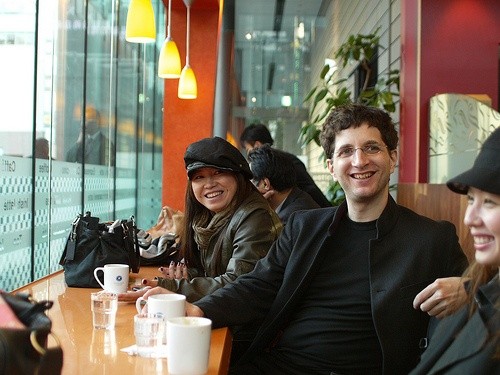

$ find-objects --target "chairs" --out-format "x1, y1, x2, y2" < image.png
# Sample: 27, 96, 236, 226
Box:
396, 181, 482, 266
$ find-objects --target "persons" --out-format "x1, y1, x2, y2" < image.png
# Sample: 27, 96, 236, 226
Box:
143, 105, 476, 375
238, 122, 334, 209
65, 108, 113, 165
246, 143, 322, 225
408, 126, 500, 375
26, 138, 56, 160
95, 136, 285, 303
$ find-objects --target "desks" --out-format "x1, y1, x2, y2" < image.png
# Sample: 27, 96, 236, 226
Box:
0, 224, 234, 375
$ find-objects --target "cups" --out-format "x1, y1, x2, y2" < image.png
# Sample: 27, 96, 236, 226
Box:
134, 314, 163, 355
166, 317, 212, 375
90, 293, 118, 329
94, 264, 129, 294
136, 294, 186, 344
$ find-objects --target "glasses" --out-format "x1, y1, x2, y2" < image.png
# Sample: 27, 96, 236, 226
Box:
333, 143, 392, 156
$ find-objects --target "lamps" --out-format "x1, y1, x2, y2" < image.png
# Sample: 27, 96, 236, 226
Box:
159, 0, 182, 80
126, 0, 156, 44
178, 0, 198, 99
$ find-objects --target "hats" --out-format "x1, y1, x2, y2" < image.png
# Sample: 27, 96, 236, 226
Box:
446, 125, 500, 196
183, 137, 254, 182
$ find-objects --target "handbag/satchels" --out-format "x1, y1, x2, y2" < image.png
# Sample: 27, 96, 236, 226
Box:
145, 205, 184, 238
136, 227, 182, 267
0, 289, 65, 375
57, 211, 142, 288
